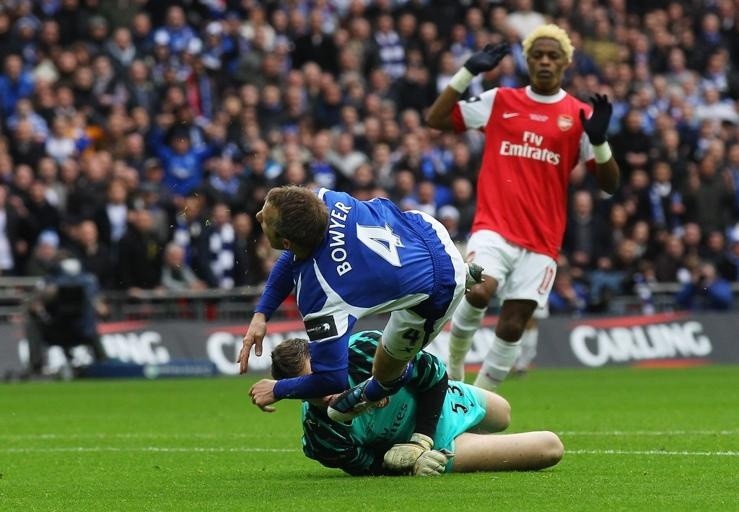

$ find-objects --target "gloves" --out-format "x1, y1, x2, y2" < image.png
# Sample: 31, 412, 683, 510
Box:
578, 92, 614, 146
463, 41, 511, 76
383, 432, 448, 477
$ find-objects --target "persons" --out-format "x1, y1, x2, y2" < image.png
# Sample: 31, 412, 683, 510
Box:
1, 2, 737, 379
231, 22, 622, 475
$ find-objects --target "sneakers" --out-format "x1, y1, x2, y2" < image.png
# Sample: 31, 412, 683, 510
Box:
466, 262, 486, 286
326, 376, 388, 422
